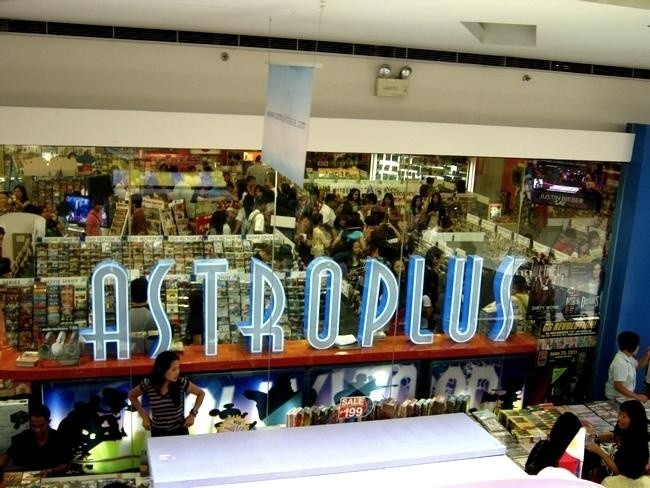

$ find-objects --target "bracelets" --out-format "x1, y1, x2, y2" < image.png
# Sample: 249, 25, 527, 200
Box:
190, 408, 198, 416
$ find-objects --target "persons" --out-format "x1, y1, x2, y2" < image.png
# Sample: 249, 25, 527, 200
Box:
484, 269, 529, 328
0, 185, 72, 237
524, 400, 650, 488
185, 289, 205, 345
131, 194, 148, 235
604, 330, 650, 402
86, 200, 104, 236
553, 227, 603, 262
157, 155, 267, 202
579, 259, 603, 295
129, 277, 157, 353
209, 173, 447, 333
0, 227, 12, 278
128, 351, 205, 436
0, 405, 74, 478
526, 270, 555, 327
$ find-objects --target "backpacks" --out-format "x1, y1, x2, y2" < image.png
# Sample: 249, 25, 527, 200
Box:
241, 212, 262, 234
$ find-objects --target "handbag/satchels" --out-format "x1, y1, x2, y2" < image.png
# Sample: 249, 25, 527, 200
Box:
437, 215, 453, 228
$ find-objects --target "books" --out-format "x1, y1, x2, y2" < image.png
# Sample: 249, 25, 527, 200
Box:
2, 470, 153, 488
472, 395, 650, 470
16, 351, 41, 367
285, 394, 471, 428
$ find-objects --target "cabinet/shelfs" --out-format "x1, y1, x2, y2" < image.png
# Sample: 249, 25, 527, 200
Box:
0, 152, 620, 351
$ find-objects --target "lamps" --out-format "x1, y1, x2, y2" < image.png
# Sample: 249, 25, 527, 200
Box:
373, 62, 411, 99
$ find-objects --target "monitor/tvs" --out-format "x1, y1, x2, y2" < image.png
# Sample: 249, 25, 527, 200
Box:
64, 193, 92, 224
